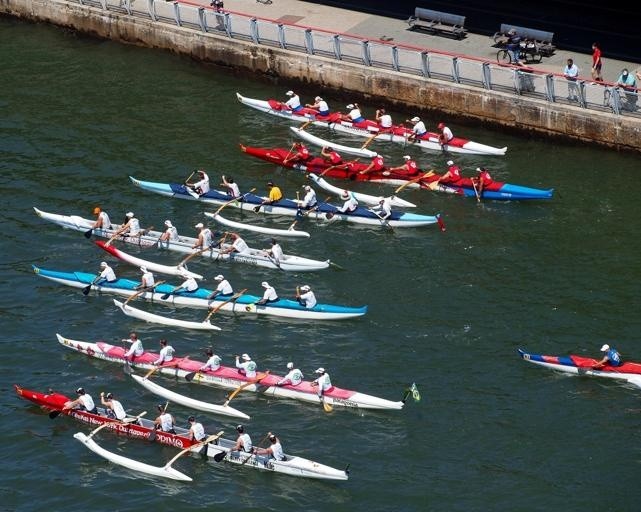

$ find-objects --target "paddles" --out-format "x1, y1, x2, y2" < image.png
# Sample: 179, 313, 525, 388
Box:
170, 164, 404, 240
255, 382, 292, 393
577, 366, 593, 374
123, 341, 133, 376
185, 368, 202, 380
312, 384, 332, 413
79, 270, 301, 326
429, 137, 447, 190
85, 215, 286, 274
49, 399, 269, 473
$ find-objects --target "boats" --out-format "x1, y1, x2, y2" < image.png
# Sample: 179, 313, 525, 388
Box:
237, 93, 508, 159
240, 142, 555, 208
31, 263, 369, 332
34, 207, 330, 280
15, 385, 349, 481
517, 348, 641, 379
129, 175, 441, 239
55, 332, 416, 422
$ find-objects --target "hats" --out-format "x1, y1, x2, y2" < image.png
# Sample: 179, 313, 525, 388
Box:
93, 208, 278, 245
130, 333, 325, 374
265, 180, 385, 202
286, 91, 444, 128
368, 152, 485, 173
508, 29, 516, 35
100, 262, 310, 292
600, 344, 609, 351
76, 388, 276, 441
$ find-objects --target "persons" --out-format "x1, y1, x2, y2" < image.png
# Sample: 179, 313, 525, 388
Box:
591, 343, 623, 370
613, 68, 639, 113
278, 363, 332, 398
200, 349, 256, 377
175, 272, 233, 298
280, 90, 329, 116
93, 261, 154, 290
122, 333, 175, 365
502, 29, 526, 67
263, 181, 358, 213
283, 142, 342, 165
406, 117, 453, 144
62, 387, 126, 420
564, 58, 581, 102
155, 404, 205, 442
185, 170, 242, 202
369, 196, 391, 220
209, 1, 225, 32
230, 425, 284, 462
161, 220, 248, 253
263, 237, 285, 260
439, 161, 493, 194
565, 380, 611, 400
591, 42, 604, 87
341, 103, 393, 128
91, 207, 141, 236
258, 282, 317, 309
360, 152, 417, 175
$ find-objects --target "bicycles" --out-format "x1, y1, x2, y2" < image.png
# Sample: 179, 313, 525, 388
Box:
497, 41, 543, 64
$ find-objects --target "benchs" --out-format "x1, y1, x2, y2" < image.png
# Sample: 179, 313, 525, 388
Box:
492, 22, 555, 57
403, 6, 468, 39
